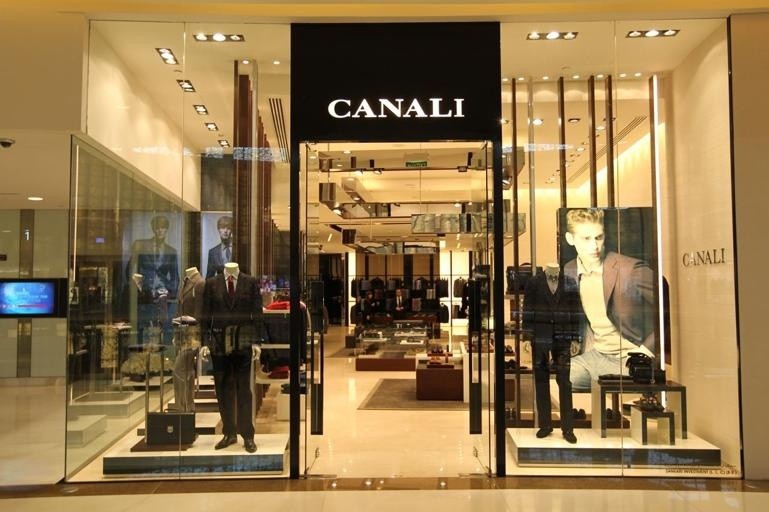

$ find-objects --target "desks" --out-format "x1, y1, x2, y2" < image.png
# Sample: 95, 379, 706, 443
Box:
460, 340, 495, 404
631, 406, 674, 445
277, 384, 306, 422
591, 378, 687, 440
416, 362, 463, 401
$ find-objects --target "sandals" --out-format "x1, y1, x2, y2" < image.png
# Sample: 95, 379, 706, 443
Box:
648, 396, 663, 411
639, 398, 654, 411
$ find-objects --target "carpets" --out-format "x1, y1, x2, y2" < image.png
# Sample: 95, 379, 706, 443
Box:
357, 376, 560, 411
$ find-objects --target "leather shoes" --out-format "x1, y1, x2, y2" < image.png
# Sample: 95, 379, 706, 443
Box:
359, 328, 379, 355
571, 409, 578, 417
503, 344, 516, 369
504, 407, 510, 419
512, 409, 515, 419
577, 409, 585, 419
429, 343, 442, 364
606, 408, 612, 419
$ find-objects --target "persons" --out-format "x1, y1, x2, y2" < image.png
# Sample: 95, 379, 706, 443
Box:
389, 288, 408, 318
520, 265, 583, 444
166, 264, 208, 411
363, 290, 374, 322
204, 215, 233, 280
197, 263, 264, 452
562, 208, 661, 382
122, 212, 177, 346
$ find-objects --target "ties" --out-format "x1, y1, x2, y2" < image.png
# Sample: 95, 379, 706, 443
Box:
226, 276, 235, 299
225, 247, 230, 259
398, 296, 400, 307
155, 244, 160, 258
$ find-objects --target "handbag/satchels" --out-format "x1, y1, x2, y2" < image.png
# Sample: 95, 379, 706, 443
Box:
633, 367, 665, 385
147, 409, 196, 447
624, 352, 651, 376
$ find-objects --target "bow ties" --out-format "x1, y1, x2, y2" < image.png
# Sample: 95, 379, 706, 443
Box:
548, 274, 557, 280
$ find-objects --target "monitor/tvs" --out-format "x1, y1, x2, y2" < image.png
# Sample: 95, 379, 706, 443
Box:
0, 278, 68, 318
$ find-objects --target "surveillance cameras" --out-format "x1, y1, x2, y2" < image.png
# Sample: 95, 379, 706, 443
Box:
0, 140, 13, 147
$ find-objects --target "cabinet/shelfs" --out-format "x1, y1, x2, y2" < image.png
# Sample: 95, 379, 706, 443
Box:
347, 311, 440, 371
250, 309, 321, 429
505, 330, 538, 428
